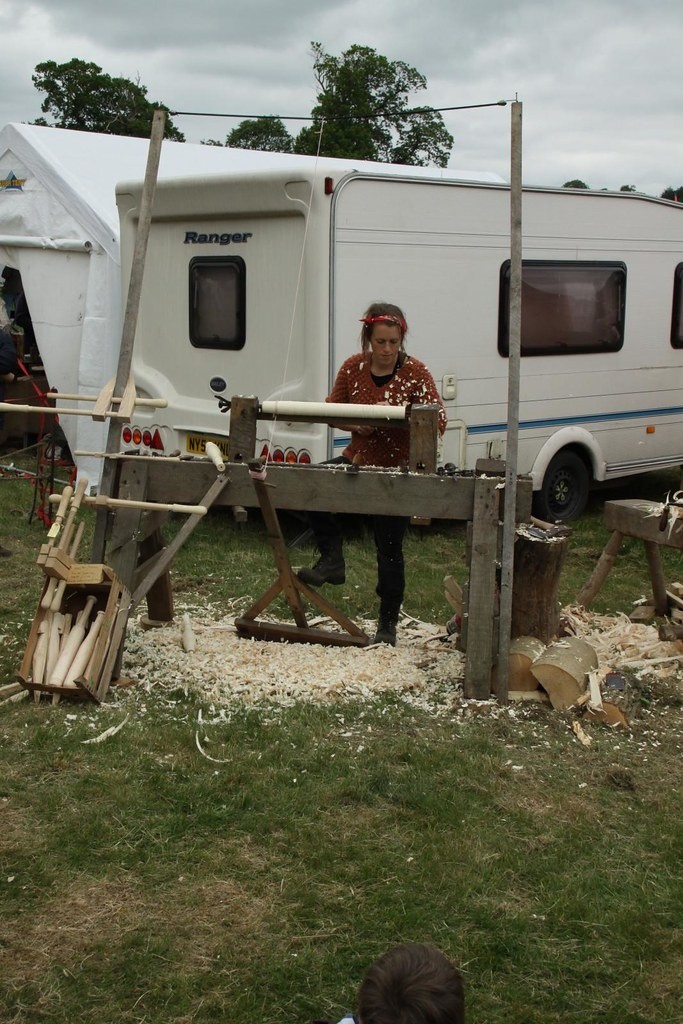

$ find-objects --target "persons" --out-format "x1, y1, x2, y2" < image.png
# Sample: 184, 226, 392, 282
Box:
297, 303, 449, 649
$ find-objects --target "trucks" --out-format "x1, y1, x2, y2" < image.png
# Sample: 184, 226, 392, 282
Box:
116, 168, 683, 530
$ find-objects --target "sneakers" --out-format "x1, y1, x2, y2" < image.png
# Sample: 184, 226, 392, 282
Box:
298, 554, 346, 587
373, 616, 396, 646
